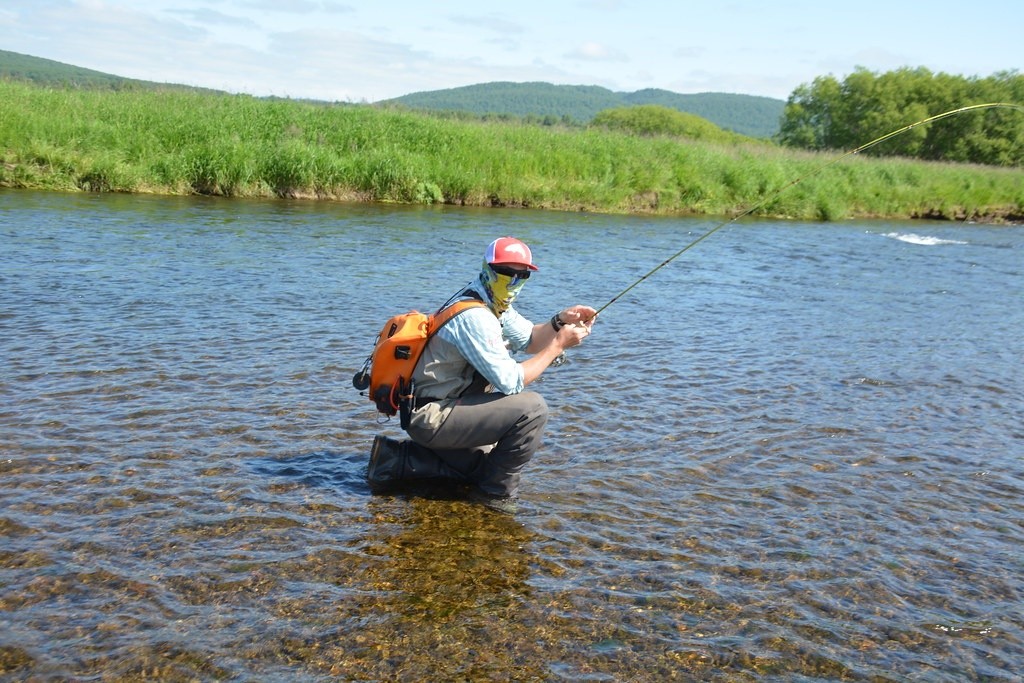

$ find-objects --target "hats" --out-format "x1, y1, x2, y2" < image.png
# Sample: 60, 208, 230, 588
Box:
485, 236, 539, 270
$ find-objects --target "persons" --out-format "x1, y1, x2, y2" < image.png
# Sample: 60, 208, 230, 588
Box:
370, 237, 599, 496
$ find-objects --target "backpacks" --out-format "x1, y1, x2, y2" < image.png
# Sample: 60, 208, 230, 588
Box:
353, 282, 493, 424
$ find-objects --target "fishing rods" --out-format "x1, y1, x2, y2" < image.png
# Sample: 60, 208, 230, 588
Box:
547, 103, 1024, 376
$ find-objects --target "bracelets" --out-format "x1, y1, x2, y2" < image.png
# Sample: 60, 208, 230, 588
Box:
551, 313, 567, 332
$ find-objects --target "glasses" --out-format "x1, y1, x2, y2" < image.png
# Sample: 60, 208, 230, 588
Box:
491, 265, 531, 279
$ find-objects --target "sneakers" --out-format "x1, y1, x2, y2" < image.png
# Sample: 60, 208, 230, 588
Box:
474, 490, 536, 517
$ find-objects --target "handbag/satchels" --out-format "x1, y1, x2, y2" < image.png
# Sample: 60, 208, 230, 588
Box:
367, 435, 496, 495
